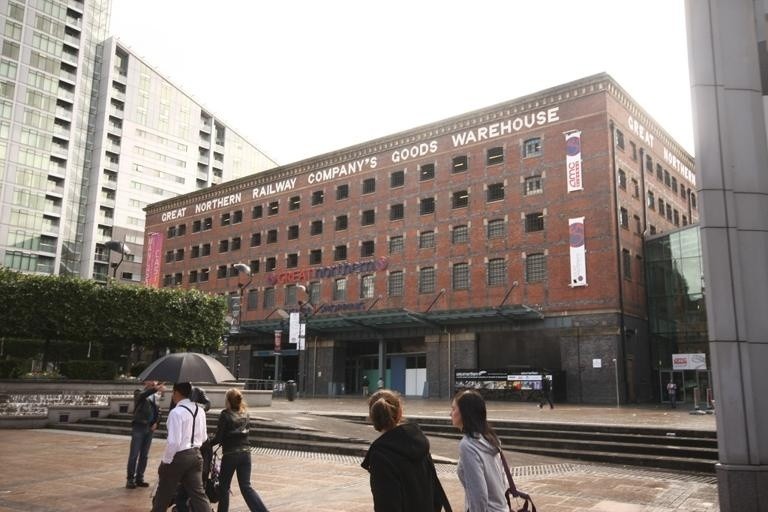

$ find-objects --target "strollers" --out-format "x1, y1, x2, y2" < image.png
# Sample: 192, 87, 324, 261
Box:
151, 437, 221, 512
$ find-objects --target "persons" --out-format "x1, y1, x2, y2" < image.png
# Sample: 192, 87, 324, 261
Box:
361, 375, 368, 398
358, 388, 452, 512
448, 390, 513, 512
537, 372, 555, 409
125, 380, 269, 512
242, 375, 286, 396
666, 379, 676, 409
376, 378, 383, 390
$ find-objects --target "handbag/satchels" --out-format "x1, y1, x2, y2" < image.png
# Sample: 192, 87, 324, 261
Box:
505, 489, 536, 512
206, 471, 225, 503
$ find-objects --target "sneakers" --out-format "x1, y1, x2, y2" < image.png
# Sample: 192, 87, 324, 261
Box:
126, 479, 135, 488
136, 480, 148, 487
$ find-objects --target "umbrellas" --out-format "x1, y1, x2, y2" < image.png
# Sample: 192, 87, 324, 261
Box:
136, 352, 236, 384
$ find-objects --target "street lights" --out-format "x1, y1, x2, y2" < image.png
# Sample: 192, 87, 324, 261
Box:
230, 264, 255, 384
111, 240, 130, 276
295, 279, 317, 400
